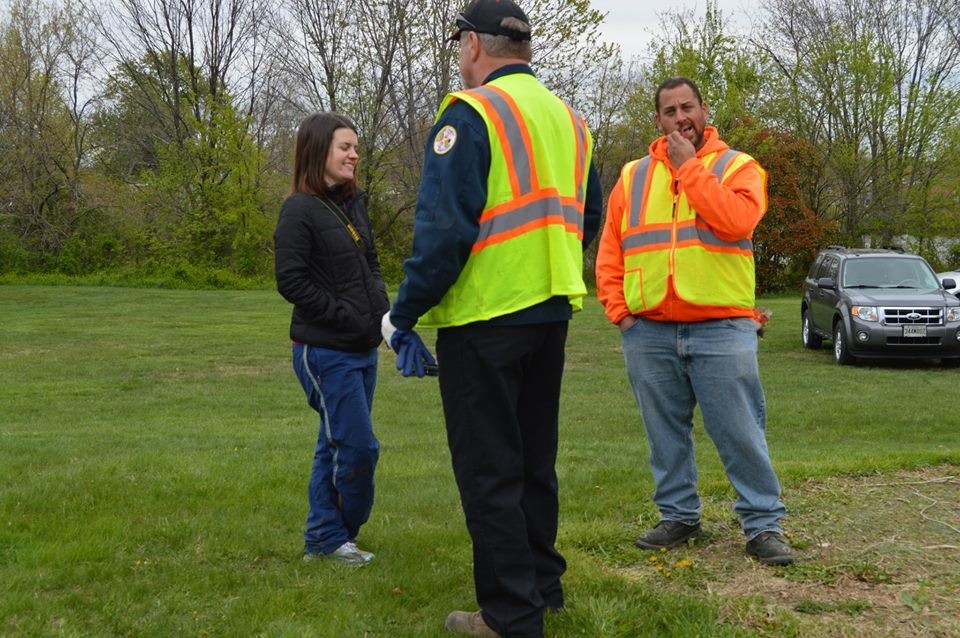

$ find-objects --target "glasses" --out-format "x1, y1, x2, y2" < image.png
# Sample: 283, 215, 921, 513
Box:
455, 12, 476, 30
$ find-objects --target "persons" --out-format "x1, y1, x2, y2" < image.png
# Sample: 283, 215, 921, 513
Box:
595, 76, 795, 565
275, 115, 390, 563
382, 0, 602, 638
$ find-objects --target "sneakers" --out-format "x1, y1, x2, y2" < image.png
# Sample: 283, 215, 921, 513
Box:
304, 541, 375, 567
747, 529, 794, 564
636, 514, 699, 549
445, 611, 500, 638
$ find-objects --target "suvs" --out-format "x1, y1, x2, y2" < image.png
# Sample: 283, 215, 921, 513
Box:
800, 244, 960, 367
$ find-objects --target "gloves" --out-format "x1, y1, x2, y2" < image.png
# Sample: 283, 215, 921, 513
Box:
380, 311, 436, 378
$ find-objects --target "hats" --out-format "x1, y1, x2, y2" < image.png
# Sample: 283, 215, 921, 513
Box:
444, 0, 532, 45
